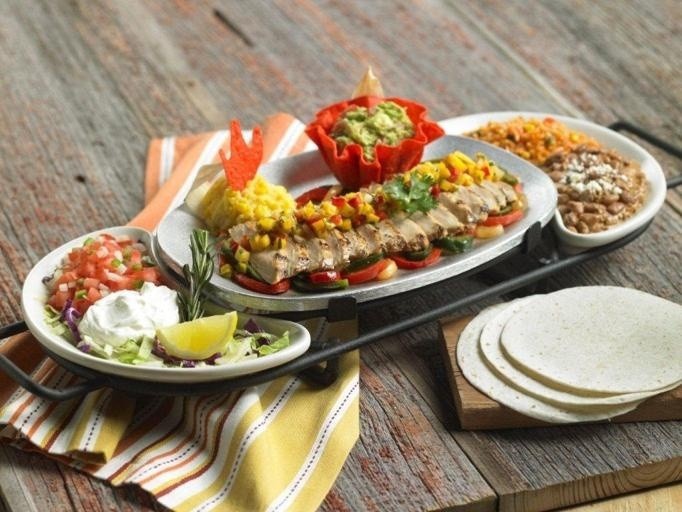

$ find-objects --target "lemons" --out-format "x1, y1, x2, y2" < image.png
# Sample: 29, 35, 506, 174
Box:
155, 311, 238, 361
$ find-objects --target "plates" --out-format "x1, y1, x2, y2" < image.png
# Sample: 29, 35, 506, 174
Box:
22, 226, 312, 383
432, 110, 667, 248
155, 132, 558, 313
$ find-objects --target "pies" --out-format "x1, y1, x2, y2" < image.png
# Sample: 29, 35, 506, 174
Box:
455, 286, 682, 425
